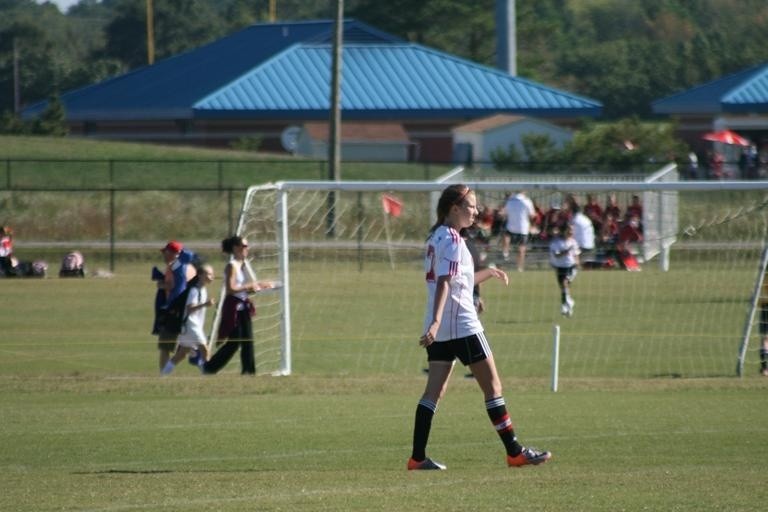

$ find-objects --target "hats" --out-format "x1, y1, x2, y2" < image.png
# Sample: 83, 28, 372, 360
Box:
161, 242, 181, 254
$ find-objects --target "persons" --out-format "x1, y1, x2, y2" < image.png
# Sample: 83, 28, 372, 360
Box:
461, 189, 641, 268
623, 139, 764, 179
202, 237, 271, 374
408, 183, 552, 470
148, 240, 198, 376
757, 266, 768, 378
159, 260, 217, 376
0, 226, 13, 277
421, 226, 484, 379
11, 251, 84, 279
550, 224, 581, 317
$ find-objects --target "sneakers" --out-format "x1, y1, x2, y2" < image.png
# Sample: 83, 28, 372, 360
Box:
507, 446, 551, 466
561, 299, 575, 318
407, 457, 445, 470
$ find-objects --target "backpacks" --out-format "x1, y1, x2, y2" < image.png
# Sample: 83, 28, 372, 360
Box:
6, 263, 31, 277
60, 253, 84, 276
31, 261, 47, 276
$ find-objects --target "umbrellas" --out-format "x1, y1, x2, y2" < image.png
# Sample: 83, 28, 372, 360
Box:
699, 127, 750, 148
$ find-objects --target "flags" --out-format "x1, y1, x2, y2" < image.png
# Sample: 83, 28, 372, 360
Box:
383, 194, 401, 218
